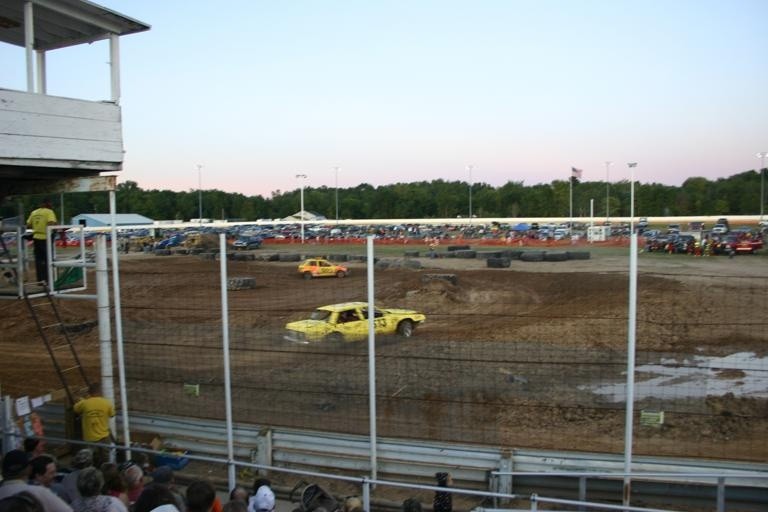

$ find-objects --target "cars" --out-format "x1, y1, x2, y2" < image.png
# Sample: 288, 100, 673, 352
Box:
279, 300, 426, 350
294, 258, 351, 280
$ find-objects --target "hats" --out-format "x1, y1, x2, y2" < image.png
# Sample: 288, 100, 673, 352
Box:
253, 486, 276, 509
154, 466, 175, 482
41, 197, 52, 203
2, 449, 30, 473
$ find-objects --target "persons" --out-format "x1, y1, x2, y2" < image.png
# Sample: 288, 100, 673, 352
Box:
67, 381, 115, 445
0, 438, 454, 512
26, 196, 58, 285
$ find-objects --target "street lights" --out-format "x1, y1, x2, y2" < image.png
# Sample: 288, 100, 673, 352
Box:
194, 163, 203, 223
623, 160, 638, 218
332, 165, 340, 220
294, 172, 309, 245
754, 150, 768, 216
